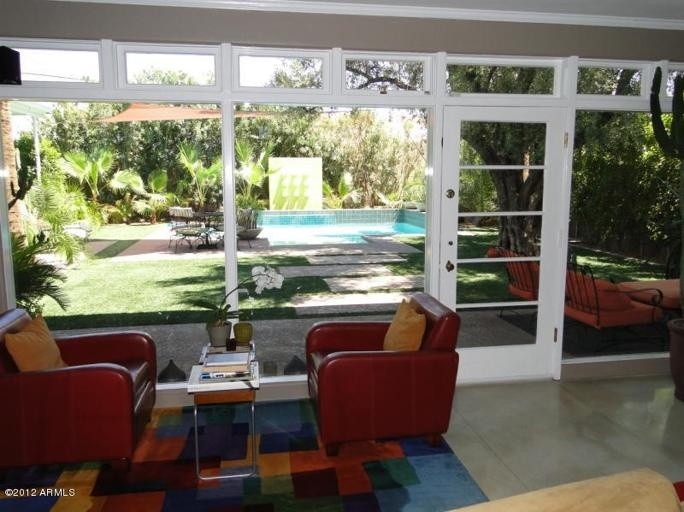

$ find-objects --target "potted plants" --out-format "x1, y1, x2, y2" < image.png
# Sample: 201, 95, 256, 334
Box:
179, 146, 219, 212
649, 60, 684, 402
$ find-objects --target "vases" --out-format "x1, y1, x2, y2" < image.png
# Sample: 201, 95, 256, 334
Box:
207, 320, 232, 346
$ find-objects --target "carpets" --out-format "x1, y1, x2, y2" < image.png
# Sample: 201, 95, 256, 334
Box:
0, 398, 489, 512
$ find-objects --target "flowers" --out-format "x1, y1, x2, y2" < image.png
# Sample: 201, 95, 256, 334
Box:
178, 261, 285, 328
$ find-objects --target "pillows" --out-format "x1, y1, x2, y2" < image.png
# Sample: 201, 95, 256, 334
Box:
382, 297, 425, 351
2, 311, 68, 373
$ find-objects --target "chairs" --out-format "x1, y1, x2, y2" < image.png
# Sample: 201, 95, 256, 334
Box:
565, 261, 664, 352
496, 246, 539, 333
167, 206, 258, 251
305, 289, 462, 457
2, 302, 156, 476
615, 244, 683, 311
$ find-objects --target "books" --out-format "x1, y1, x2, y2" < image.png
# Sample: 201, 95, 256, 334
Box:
185, 340, 260, 394
